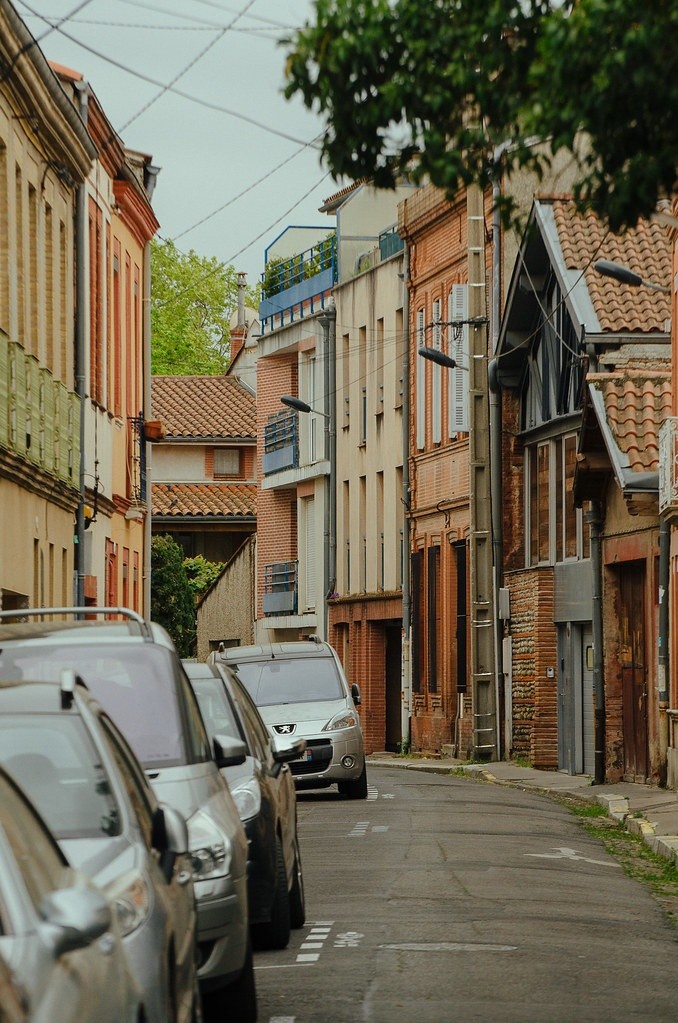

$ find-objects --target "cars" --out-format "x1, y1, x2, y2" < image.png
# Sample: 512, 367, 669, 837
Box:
0, 767, 146, 1023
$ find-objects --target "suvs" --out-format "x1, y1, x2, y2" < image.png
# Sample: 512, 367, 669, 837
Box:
207, 634, 369, 799
180, 659, 305, 949
0, 670, 208, 1023
0, 605, 258, 1023
